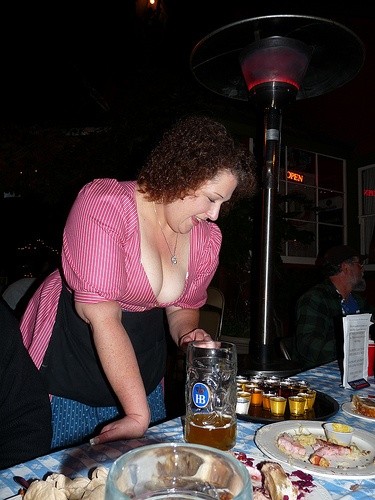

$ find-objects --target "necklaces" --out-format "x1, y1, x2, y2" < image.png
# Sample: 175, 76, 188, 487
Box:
152, 201, 179, 265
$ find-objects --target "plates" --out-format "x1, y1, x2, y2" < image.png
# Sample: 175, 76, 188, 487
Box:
255, 420, 375, 480
342, 397, 375, 421
229, 452, 332, 500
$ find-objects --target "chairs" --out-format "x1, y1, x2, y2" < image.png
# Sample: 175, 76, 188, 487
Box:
241, 266, 306, 370
197, 287, 226, 341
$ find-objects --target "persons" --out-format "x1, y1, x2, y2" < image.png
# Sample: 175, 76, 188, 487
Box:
295, 244, 375, 370
0, 296, 53, 471
20, 118, 257, 453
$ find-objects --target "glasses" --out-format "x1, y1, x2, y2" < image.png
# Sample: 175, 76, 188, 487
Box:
353, 261, 367, 271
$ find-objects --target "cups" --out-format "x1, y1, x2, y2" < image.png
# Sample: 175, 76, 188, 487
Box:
235, 374, 316, 416
104, 442, 253, 500
184, 340, 237, 451
321, 423, 355, 446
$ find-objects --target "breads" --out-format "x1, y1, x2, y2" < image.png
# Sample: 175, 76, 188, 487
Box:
260, 462, 298, 500
352, 394, 375, 417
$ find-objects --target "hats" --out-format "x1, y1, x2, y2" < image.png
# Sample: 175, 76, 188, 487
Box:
320, 245, 368, 266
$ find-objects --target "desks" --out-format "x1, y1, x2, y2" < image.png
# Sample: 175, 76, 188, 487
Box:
0, 356, 375, 500
